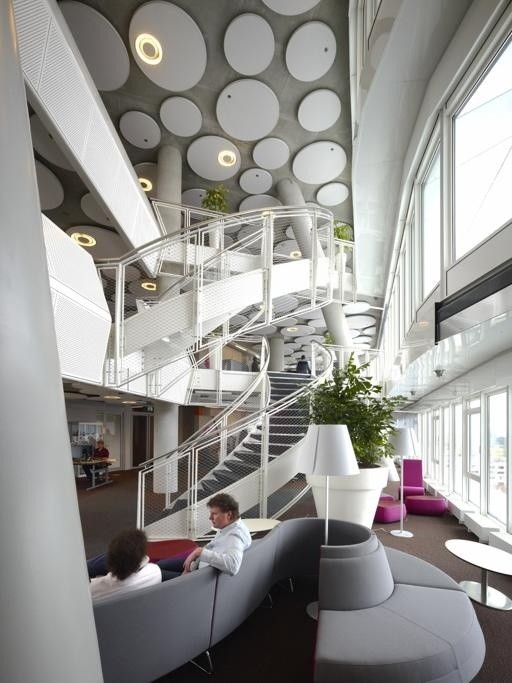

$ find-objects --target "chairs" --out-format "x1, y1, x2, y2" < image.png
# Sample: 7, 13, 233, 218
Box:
399, 459, 424, 504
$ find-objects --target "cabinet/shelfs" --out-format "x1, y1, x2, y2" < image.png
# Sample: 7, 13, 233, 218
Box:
70, 422, 103, 446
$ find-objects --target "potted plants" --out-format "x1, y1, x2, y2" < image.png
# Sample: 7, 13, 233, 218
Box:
333, 220, 354, 272
297, 351, 409, 530
200, 183, 231, 248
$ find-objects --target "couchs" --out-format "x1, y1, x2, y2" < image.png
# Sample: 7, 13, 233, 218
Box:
92, 518, 486, 683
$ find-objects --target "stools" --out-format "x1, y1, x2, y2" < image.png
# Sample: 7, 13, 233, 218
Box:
374, 493, 447, 524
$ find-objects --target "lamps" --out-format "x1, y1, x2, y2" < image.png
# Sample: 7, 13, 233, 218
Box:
299, 424, 361, 621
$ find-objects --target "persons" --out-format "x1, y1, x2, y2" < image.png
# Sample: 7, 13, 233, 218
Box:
156, 493, 252, 581
82, 440, 109, 480
86, 528, 162, 601
296, 355, 311, 374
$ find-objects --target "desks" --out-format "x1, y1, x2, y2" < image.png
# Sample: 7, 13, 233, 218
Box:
73, 458, 116, 491
